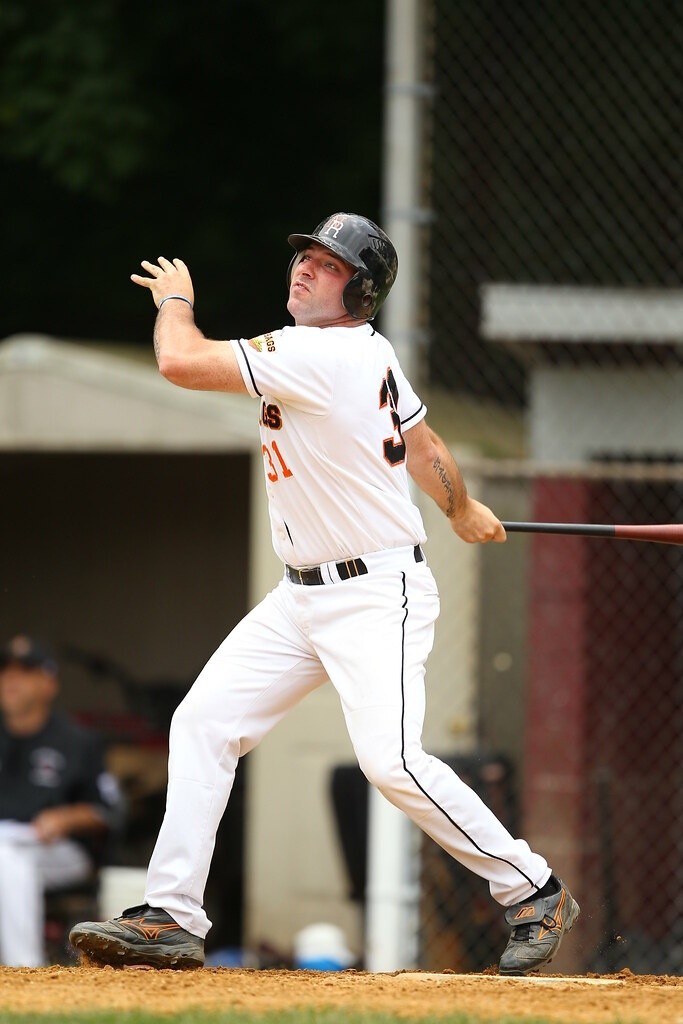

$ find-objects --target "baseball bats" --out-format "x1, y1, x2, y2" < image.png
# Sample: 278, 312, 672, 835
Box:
501, 521, 683, 546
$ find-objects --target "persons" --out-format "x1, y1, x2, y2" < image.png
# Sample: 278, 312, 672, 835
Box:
73, 213, 581, 975
0, 631, 126, 966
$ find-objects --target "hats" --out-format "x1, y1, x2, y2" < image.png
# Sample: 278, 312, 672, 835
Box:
0, 624, 68, 676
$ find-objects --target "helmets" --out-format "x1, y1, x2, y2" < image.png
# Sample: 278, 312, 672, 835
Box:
289, 214, 400, 319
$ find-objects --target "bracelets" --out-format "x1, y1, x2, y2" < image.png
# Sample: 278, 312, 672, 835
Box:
159, 294, 193, 309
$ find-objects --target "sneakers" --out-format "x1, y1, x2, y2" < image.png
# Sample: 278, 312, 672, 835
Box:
499, 878, 581, 975
68, 904, 206, 968
